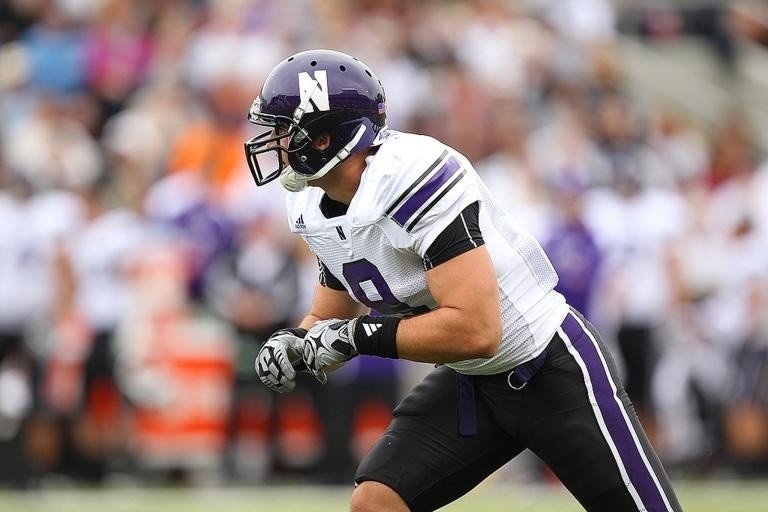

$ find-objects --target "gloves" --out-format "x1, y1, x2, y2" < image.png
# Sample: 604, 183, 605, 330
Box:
253, 328, 303, 394
302, 317, 357, 384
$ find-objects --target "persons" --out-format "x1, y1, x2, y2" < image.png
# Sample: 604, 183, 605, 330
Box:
245, 49, 680, 512
1, 3, 768, 485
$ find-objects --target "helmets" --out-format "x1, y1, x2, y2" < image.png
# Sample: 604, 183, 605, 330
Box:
243, 48, 388, 192
